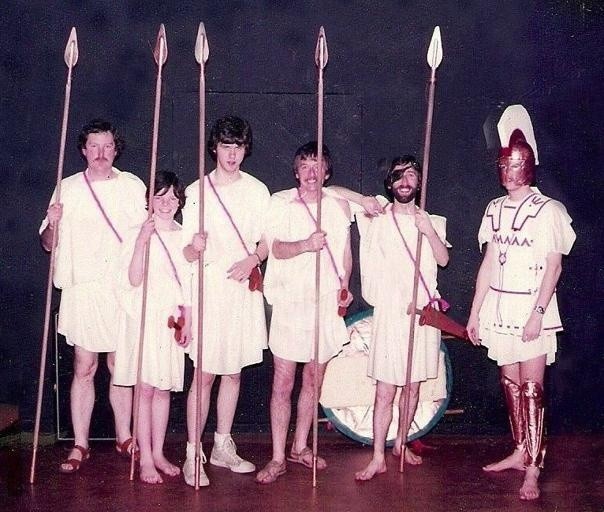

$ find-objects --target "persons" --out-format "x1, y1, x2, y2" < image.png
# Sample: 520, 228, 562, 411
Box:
256, 140, 352, 487
38, 121, 151, 476
466, 143, 577, 501
325, 154, 452, 482
178, 116, 270, 487
125, 170, 192, 486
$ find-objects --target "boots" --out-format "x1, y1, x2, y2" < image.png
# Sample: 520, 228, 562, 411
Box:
210, 431, 256, 474
182, 441, 210, 487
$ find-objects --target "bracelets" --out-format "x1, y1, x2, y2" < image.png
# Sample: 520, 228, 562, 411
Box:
48, 223, 55, 231
533, 305, 547, 315
253, 252, 263, 266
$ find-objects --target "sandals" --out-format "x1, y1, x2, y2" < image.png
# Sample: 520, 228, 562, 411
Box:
114, 436, 140, 462
255, 459, 288, 484
59, 445, 93, 474
288, 447, 327, 470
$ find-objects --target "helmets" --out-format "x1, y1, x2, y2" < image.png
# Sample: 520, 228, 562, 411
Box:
498, 129, 535, 186
